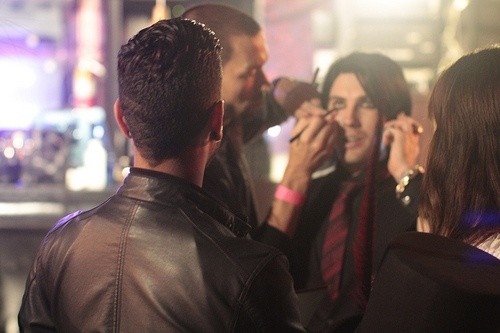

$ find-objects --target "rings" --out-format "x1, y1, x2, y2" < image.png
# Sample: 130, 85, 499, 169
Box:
412, 123, 423, 137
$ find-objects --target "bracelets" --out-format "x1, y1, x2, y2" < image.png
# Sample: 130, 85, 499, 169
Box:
273, 182, 306, 207
395, 164, 425, 198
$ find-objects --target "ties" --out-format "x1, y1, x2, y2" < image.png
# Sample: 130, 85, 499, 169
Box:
226, 142, 251, 210
319, 179, 365, 302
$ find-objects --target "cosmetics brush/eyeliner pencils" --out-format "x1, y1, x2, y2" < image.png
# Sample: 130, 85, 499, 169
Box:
287, 106, 338, 145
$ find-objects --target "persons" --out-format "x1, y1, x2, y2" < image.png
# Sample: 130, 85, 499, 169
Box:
290, 52, 425, 333
17, 17, 304, 333
357, 48, 500, 333
178, 5, 341, 255
